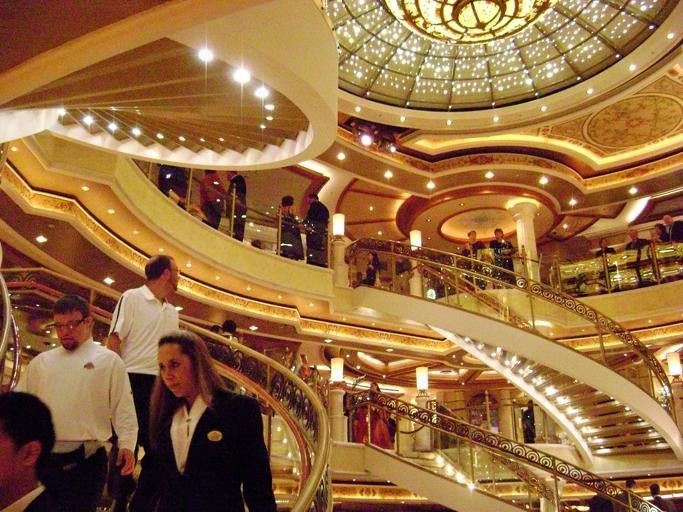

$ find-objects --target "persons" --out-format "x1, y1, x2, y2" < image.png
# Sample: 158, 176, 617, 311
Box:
612, 480, 642, 511
569, 215, 682, 295
126, 333, 278, 511
205, 316, 400, 452
589, 479, 612, 511
521, 400, 536, 444
0, 390, 68, 512
106, 256, 181, 512
644, 484, 676, 511
362, 229, 518, 295
12, 294, 138, 511
155, 162, 329, 268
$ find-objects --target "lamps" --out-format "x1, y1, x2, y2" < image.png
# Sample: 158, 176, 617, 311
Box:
415, 366, 429, 390
332, 213, 346, 236
409, 230, 422, 252
382, 0, 557, 45
330, 356, 344, 382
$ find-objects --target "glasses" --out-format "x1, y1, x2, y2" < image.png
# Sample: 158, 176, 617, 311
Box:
53, 319, 84, 329
